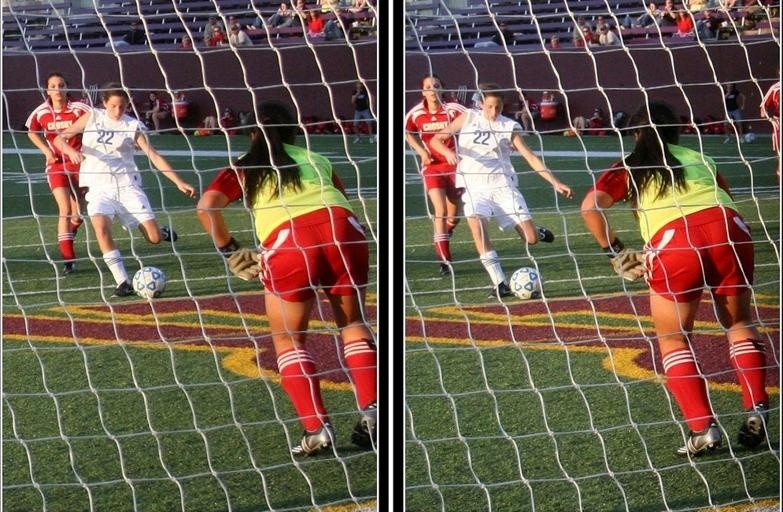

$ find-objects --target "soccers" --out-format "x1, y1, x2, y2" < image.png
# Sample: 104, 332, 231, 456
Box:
510, 268, 542, 300
132, 265, 168, 298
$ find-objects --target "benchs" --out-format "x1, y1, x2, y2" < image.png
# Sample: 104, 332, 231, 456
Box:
2, 0, 780, 54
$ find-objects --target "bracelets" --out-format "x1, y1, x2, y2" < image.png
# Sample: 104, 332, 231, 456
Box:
218, 237, 239, 260
603, 237, 624, 258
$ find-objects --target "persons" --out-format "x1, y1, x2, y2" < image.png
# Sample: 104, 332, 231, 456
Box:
199, 98, 378, 457
404, 74, 468, 276
431, 84, 574, 299
53, 84, 197, 298
581, 100, 768, 456
25, 72, 92, 274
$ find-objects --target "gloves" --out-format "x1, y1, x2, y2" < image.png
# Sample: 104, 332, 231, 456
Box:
227, 246, 263, 281
610, 246, 646, 281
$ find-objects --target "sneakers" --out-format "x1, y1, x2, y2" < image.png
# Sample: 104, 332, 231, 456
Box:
488, 282, 510, 299
440, 264, 450, 276
160, 226, 177, 242
116, 280, 132, 296
64, 262, 75, 274
352, 403, 377, 445
292, 423, 336, 456
738, 401, 769, 447
677, 422, 722, 458
536, 226, 554, 242
